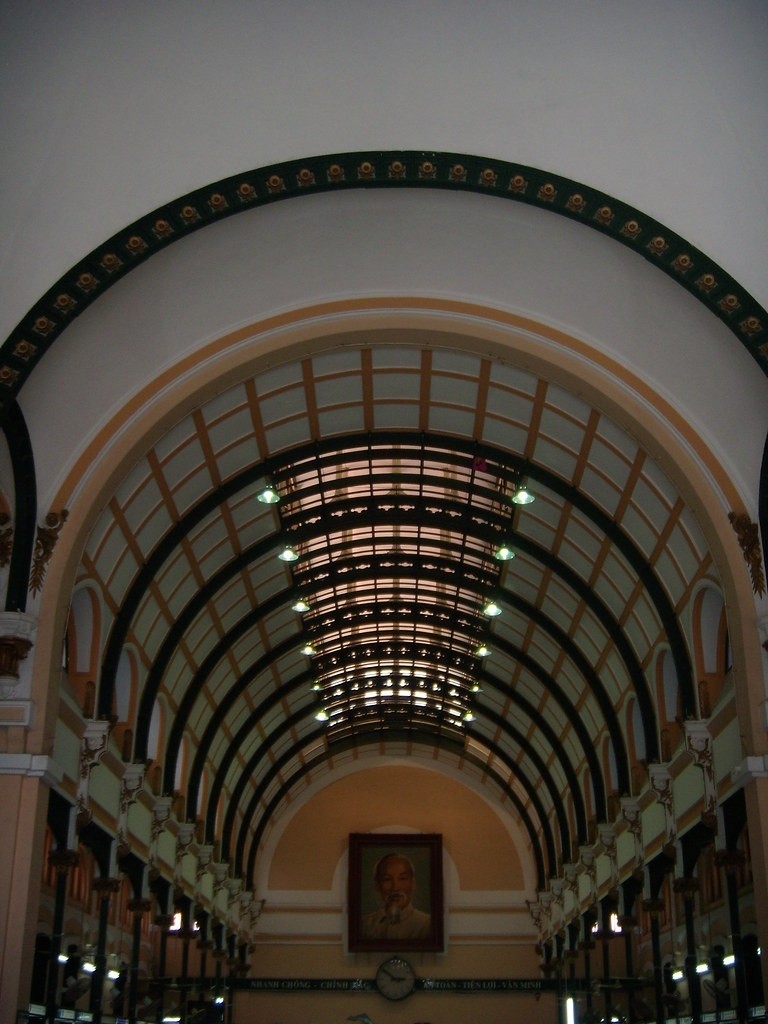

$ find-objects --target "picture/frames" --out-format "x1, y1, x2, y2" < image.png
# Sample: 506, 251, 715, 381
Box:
346, 833, 446, 954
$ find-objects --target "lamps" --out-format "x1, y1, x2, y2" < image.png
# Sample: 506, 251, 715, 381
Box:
301, 641, 317, 656
310, 678, 323, 691
463, 706, 477, 722
315, 704, 330, 722
469, 678, 483, 694
278, 540, 300, 563
512, 472, 535, 506
291, 595, 311, 612
257, 480, 281, 505
474, 633, 492, 658
482, 592, 503, 618
495, 533, 515, 561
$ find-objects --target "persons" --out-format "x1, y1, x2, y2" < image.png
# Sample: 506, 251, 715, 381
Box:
360, 851, 432, 938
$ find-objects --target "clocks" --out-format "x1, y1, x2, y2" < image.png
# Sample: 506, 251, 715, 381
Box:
374, 959, 418, 1001
